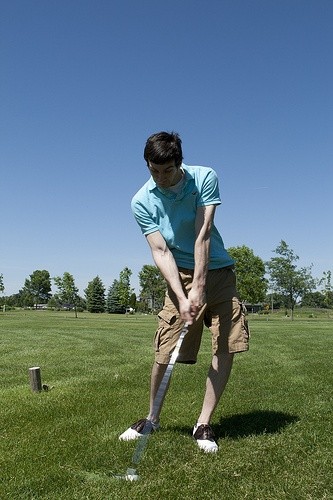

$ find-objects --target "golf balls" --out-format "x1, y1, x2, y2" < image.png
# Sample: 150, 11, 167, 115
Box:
127, 470, 139, 484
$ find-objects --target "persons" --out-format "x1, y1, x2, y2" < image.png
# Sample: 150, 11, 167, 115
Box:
119, 131, 249, 453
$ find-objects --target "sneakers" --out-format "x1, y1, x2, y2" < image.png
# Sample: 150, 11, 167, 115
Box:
119, 419, 161, 442
193, 424, 219, 453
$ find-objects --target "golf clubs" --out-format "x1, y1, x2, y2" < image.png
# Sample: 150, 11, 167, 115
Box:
130, 326, 190, 469
